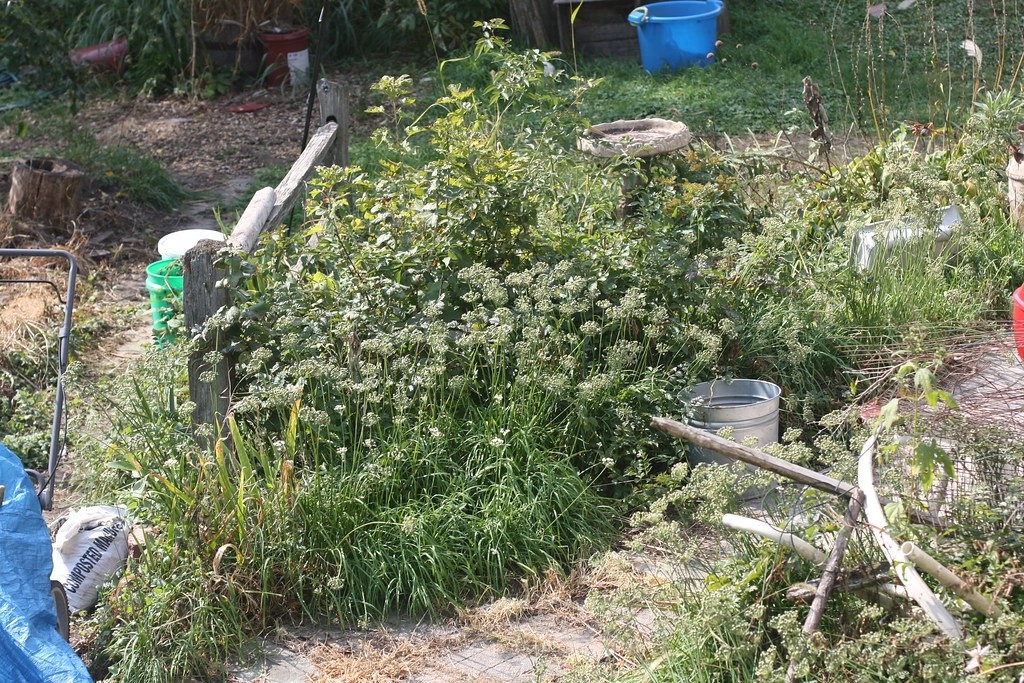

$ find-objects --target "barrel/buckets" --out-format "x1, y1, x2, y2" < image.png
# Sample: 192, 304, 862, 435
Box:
677, 379, 781, 501
69, 35, 132, 75
259, 23, 310, 95
157, 229, 230, 260
145, 258, 184, 349
627, 0, 725, 76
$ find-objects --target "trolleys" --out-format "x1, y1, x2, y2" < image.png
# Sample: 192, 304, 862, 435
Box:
0, 248, 79, 645
